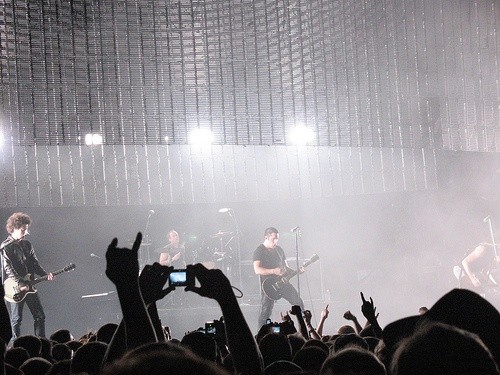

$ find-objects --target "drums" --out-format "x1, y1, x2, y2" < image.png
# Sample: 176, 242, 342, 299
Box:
216, 258, 232, 274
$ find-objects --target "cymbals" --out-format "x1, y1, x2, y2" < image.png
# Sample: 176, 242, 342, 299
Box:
209, 231, 228, 239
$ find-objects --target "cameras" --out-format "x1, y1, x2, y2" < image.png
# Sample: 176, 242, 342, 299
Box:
169, 269, 195, 286
205, 323, 216, 337
266, 319, 271, 324
272, 325, 282, 333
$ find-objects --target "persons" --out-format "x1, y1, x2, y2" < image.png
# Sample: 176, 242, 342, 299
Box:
159, 230, 195, 284
250, 225, 316, 326
0, 214, 61, 347
0, 230, 499, 375
454, 236, 499, 302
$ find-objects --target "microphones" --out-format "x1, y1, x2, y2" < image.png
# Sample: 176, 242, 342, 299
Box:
484, 216, 490, 222
91, 253, 101, 259
291, 226, 299, 232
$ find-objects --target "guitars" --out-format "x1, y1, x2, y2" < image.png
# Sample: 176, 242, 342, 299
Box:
3, 261, 77, 304
261, 254, 320, 301
